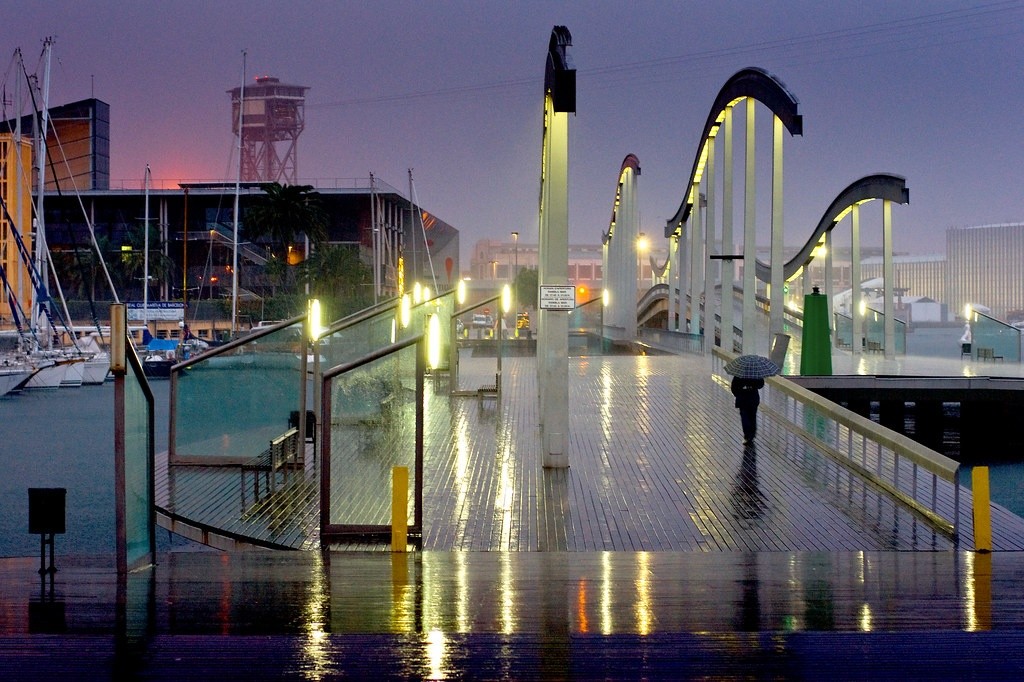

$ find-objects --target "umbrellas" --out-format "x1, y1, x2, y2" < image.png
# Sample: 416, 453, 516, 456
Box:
723, 353, 780, 378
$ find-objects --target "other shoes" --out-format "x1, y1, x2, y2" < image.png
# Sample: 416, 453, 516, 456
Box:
742, 439, 747, 444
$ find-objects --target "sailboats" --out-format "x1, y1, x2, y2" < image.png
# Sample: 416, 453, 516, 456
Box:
0, 32, 128, 395
141, 46, 349, 375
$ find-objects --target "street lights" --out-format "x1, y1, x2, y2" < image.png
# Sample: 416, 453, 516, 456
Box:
509, 230, 521, 339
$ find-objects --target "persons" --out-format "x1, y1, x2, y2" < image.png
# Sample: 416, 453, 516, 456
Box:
961, 321, 972, 341
731, 376, 764, 444
